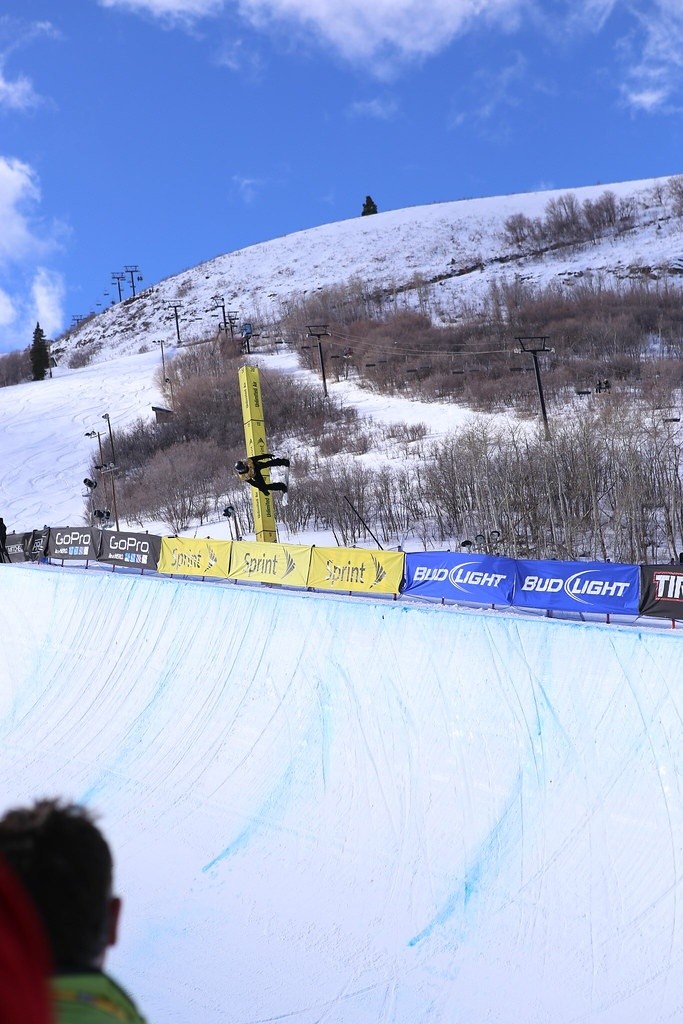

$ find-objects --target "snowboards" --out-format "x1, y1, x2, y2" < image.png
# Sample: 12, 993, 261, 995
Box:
281, 445, 292, 506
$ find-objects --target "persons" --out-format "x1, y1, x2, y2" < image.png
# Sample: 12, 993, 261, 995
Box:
0, 518, 7, 563
0, 796, 146, 1024
596, 379, 612, 394
235, 453, 290, 496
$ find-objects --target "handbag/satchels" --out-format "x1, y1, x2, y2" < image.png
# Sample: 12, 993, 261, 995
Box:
260, 488, 271, 496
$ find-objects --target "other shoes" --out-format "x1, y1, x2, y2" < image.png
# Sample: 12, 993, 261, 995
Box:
283, 458, 290, 468
281, 483, 287, 493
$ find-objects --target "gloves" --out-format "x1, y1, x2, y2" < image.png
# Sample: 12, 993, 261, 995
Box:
269, 453, 276, 460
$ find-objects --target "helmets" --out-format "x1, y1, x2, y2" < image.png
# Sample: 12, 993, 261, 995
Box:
235, 461, 247, 472
